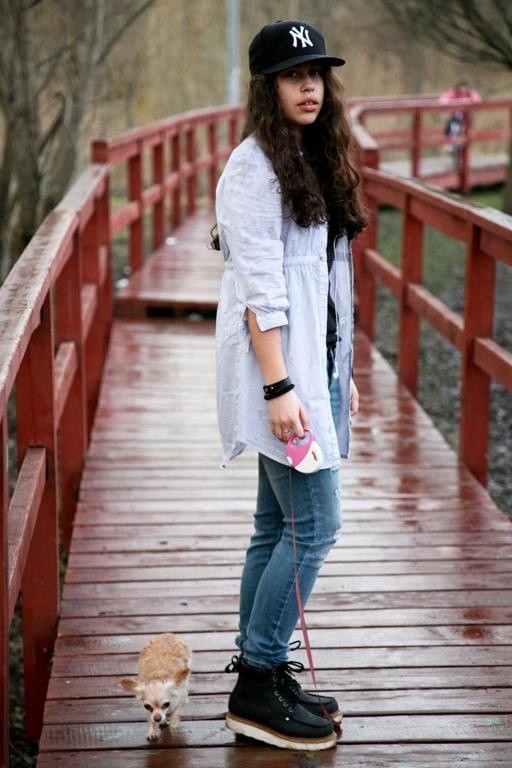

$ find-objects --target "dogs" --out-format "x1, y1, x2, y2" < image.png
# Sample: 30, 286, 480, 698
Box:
120, 633, 192, 741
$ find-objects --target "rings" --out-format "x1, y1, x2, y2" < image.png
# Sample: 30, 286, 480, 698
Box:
281, 429, 290, 433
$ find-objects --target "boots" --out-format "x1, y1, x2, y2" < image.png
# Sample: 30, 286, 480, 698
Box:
225, 658, 342, 751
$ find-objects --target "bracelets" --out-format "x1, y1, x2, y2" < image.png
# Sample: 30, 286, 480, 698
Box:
264, 383, 296, 401
262, 376, 292, 394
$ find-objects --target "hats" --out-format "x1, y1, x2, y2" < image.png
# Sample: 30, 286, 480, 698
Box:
249, 21, 345, 75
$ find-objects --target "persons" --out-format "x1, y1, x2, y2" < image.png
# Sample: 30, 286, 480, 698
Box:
435, 79, 481, 171
210, 21, 367, 753
